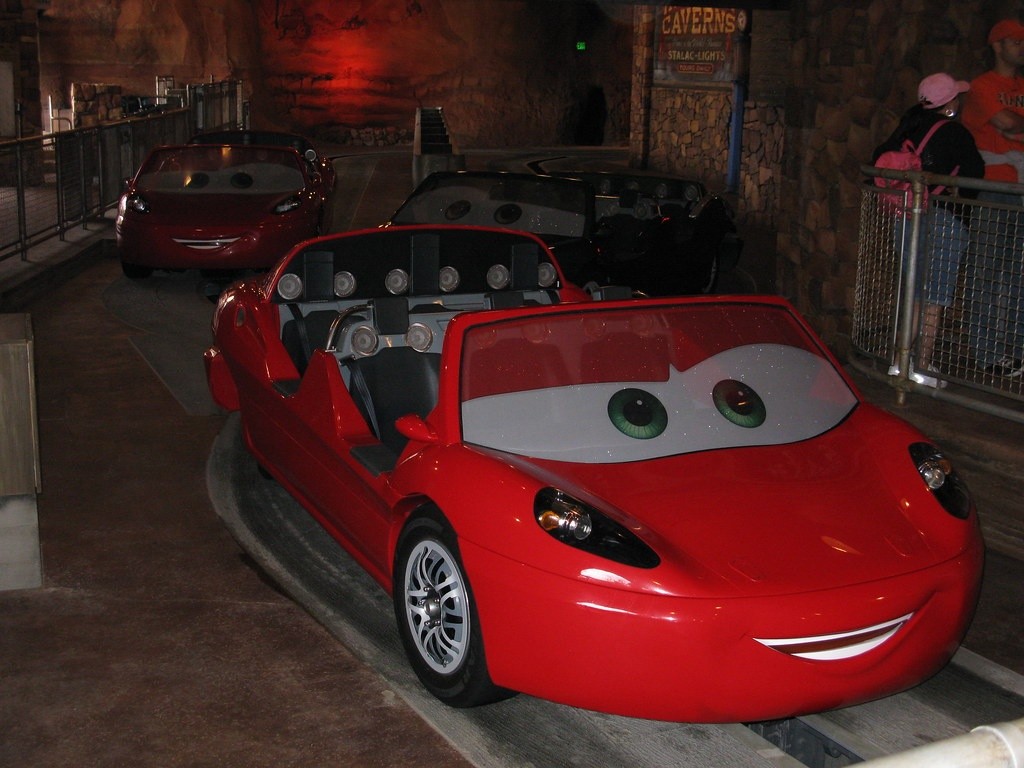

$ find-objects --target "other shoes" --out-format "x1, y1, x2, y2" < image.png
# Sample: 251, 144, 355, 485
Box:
986, 356, 1024, 378
909, 365, 947, 390
888, 365, 913, 376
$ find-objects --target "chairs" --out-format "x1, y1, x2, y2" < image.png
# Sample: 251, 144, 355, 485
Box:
348, 346, 442, 455
593, 213, 647, 238
281, 310, 367, 393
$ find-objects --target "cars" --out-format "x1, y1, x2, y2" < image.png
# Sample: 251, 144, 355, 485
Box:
188, 131, 337, 195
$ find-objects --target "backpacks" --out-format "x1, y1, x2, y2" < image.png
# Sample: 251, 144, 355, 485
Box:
871, 118, 961, 218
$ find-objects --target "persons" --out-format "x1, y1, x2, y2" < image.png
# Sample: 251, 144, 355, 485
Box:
960, 18, 1024, 378
870, 72, 986, 388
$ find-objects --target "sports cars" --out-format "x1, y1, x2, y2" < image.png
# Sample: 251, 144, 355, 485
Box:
200, 224, 986, 728
115, 144, 331, 295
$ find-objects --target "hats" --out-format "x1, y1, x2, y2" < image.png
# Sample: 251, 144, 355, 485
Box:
918, 73, 972, 109
989, 19, 1024, 42
